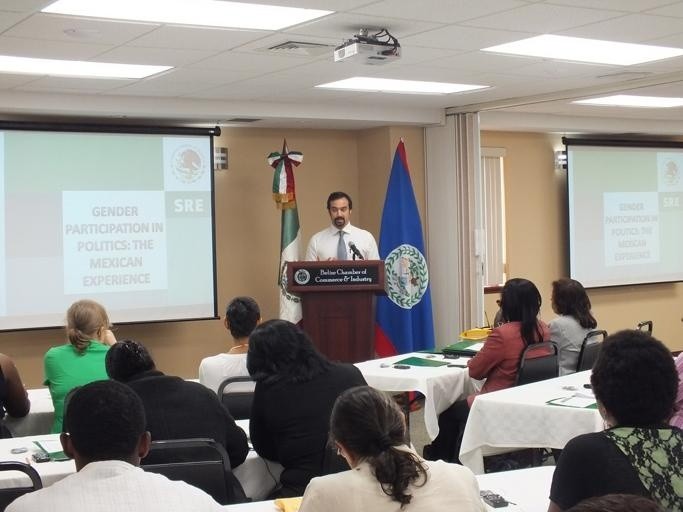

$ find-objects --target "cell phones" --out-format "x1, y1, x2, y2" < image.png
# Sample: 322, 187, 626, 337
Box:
483, 495, 508, 508
31, 451, 50, 463
394, 366, 410, 369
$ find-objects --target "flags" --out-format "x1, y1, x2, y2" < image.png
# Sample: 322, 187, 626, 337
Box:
373, 140, 434, 358
269, 139, 302, 322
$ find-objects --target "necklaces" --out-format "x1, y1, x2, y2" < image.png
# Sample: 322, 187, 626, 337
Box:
228, 343, 249, 351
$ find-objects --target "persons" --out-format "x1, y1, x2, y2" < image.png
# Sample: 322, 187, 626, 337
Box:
44, 299, 117, 436
548, 328, 683, 512
199, 296, 263, 393
6, 378, 222, 511
298, 386, 486, 510
546, 277, 598, 377
306, 194, 379, 262
246, 318, 370, 501
435, 276, 553, 443
1, 353, 30, 440
104, 338, 249, 469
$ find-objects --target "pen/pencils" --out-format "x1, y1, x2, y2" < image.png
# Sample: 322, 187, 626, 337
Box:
447, 364, 467, 368
485, 311, 490, 328
25, 457, 31, 466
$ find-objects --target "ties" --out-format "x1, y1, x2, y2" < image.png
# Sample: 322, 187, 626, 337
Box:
337, 231, 347, 260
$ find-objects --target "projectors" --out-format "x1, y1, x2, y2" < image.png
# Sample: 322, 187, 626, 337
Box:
334, 37, 401, 67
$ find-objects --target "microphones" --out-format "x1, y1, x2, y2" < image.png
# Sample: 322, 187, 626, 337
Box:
349, 241, 364, 260
